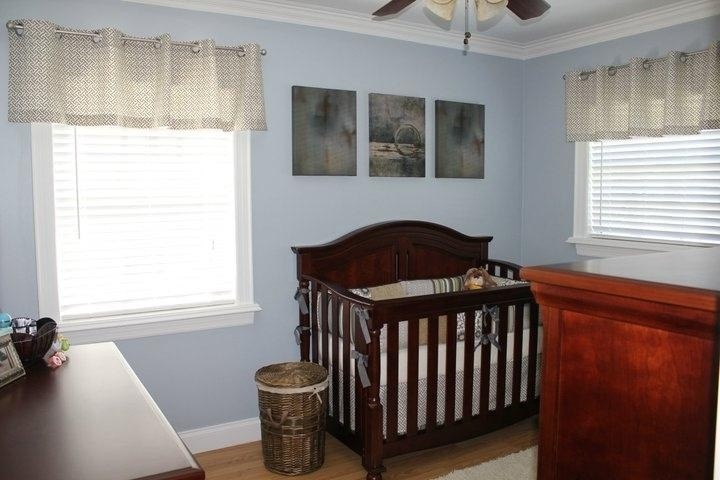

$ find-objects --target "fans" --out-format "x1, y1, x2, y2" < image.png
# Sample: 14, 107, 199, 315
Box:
372, 0, 551, 21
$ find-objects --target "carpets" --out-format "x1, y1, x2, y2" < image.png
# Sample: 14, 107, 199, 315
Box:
434, 445, 538, 479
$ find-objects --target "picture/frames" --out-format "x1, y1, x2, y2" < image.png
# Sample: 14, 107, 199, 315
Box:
0, 333, 26, 389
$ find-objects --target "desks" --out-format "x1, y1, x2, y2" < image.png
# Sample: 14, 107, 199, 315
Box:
519, 245, 720, 479
0, 341, 206, 480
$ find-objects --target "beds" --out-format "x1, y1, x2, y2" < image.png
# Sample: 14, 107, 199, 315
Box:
289, 220, 539, 479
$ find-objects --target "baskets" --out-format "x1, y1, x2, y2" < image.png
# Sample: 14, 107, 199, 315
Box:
10, 317, 58, 362
